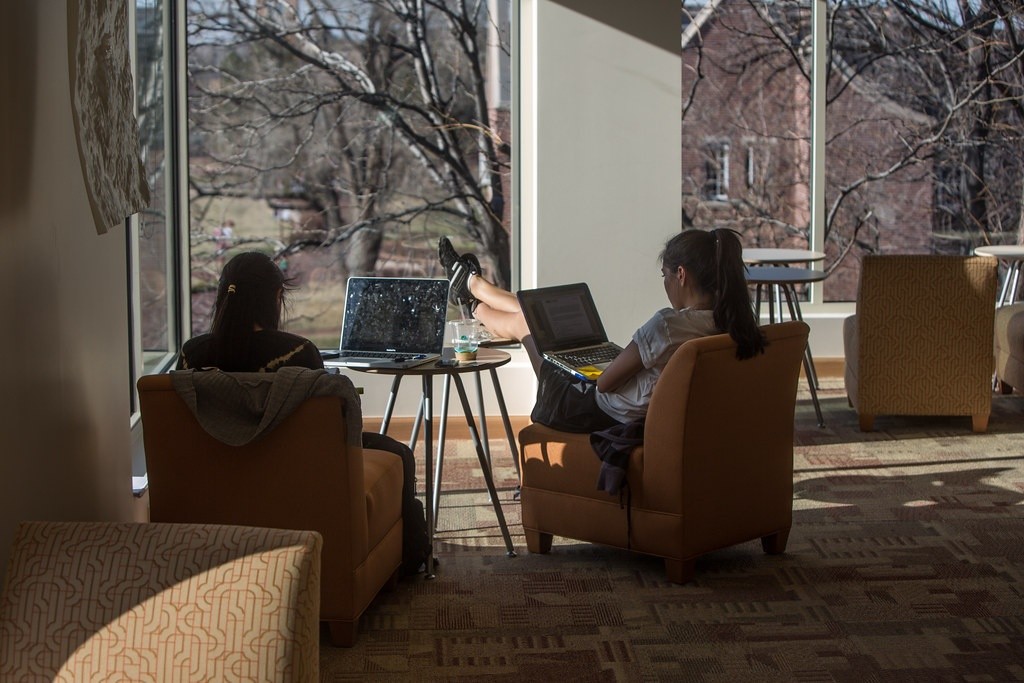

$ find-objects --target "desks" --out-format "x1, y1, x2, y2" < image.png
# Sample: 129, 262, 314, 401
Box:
741, 246, 827, 391
443, 319, 520, 498
348, 347, 517, 560
974, 245, 1024, 392
743, 266, 828, 428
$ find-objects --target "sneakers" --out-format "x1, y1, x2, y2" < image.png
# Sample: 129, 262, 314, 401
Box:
460, 253, 482, 319
438, 235, 477, 307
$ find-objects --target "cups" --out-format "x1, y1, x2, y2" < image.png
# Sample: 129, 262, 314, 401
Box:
447, 319, 481, 360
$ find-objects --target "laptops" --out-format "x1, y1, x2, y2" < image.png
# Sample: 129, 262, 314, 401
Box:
319, 277, 450, 368
517, 282, 625, 382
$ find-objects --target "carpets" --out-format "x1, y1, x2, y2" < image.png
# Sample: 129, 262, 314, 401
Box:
362, 414, 532, 494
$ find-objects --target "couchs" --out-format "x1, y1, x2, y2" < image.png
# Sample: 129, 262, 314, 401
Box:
992, 262, 1024, 395
523, 321, 811, 580
136, 373, 404, 649
842, 255, 998, 432
0, 519, 323, 683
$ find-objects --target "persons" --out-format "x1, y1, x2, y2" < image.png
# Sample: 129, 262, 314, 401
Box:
174, 251, 429, 584
439, 228, 770, 433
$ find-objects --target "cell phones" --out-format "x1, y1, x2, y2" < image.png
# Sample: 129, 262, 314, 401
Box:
320, 351, 339, 360
435, 360, 460, 368
